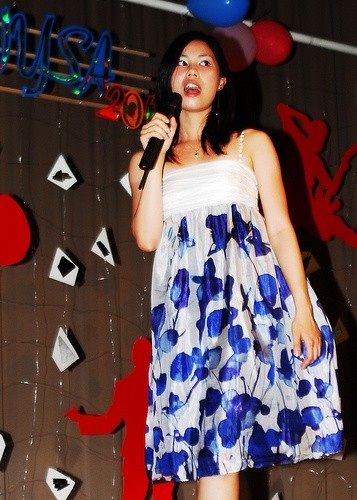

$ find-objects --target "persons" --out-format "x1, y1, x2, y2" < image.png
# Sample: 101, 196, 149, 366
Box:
129, 31, 344, 500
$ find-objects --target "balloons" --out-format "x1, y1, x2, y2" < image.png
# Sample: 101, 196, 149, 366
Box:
251, 21, 291, 64
211, 22, 257, 71
187, 0, 249, 27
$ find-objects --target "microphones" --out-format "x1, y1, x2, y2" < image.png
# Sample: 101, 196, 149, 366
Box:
139, 92, 181, 170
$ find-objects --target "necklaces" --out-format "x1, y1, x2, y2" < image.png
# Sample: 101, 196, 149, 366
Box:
179, 138, 209, 159
